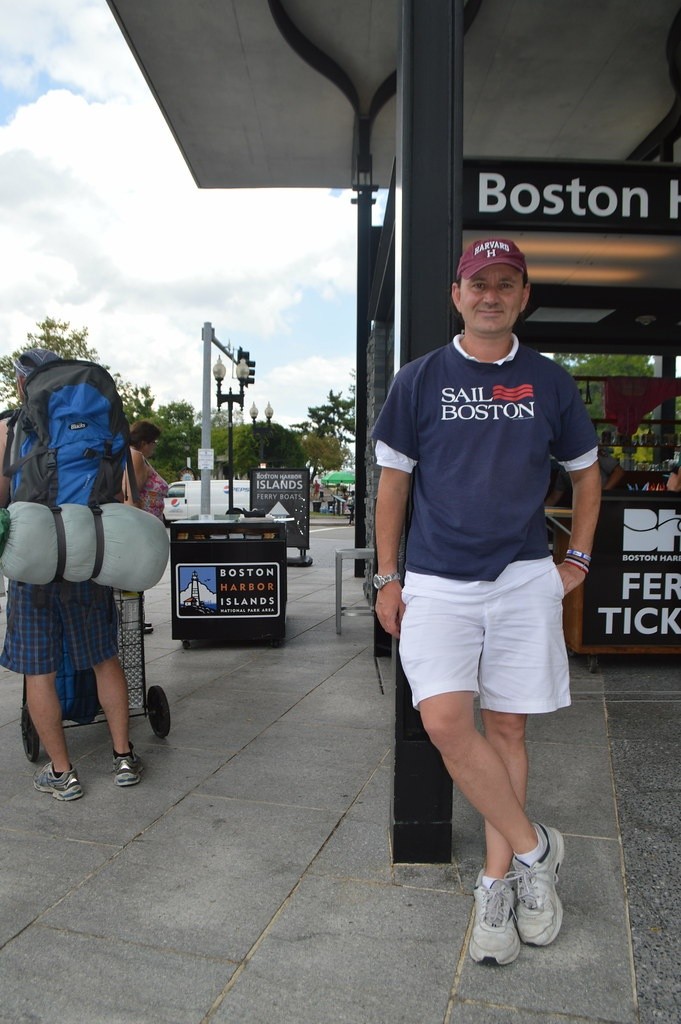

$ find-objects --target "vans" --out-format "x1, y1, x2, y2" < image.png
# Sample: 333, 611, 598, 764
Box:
161, 480, 252, 526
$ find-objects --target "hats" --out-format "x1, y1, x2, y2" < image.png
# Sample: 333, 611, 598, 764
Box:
456, 238, 527, 281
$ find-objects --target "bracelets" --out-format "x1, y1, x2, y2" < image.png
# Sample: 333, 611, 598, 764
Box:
564, 550, 592, 574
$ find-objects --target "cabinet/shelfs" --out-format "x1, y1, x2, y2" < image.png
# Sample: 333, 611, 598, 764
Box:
591, 418, 681, 489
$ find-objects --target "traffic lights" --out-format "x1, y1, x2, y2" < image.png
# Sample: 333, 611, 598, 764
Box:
241, 351, 255, 388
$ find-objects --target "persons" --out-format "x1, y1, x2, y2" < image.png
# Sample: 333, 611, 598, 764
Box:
0, 349, 168, 801
313, 480, 320, 501
371, 238, 600, 965
546, 453, 626, 508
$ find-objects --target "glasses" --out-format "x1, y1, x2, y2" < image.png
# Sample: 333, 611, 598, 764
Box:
151, 440, 158, 445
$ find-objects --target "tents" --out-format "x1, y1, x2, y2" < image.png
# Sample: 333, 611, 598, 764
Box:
321, 472, 356, 499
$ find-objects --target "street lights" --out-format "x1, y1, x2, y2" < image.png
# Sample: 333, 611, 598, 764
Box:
213, 354, 250, 514
250, 400, 273, 468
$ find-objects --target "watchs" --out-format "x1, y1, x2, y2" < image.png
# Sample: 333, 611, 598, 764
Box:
373, 573, 401, 589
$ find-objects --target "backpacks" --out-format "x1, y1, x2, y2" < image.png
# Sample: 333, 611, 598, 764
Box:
3, 359, 139, 508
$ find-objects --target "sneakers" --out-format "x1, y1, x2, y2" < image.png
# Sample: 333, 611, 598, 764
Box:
112, 741, 144, 786
32, 761, 84, 801
503, 821, 564, 946
469, 868, 520, 965
